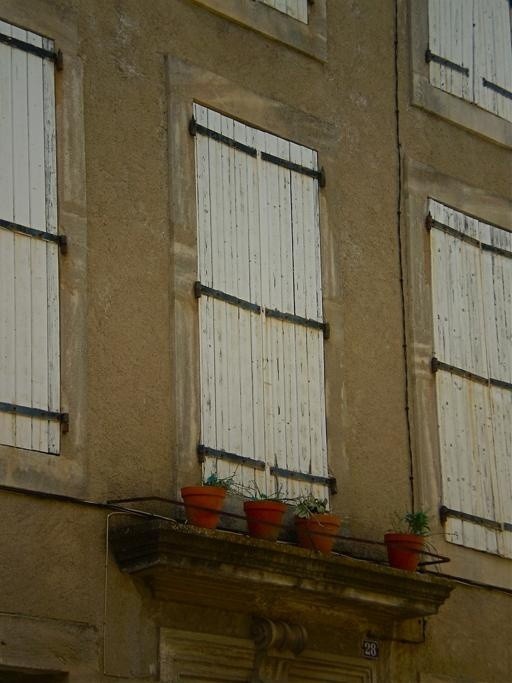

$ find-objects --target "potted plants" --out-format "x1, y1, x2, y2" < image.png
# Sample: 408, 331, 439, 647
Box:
384, 510, 432, 571
244, 475, 288, 542
294, 491, 342, 553
181, 470, 236, 530
